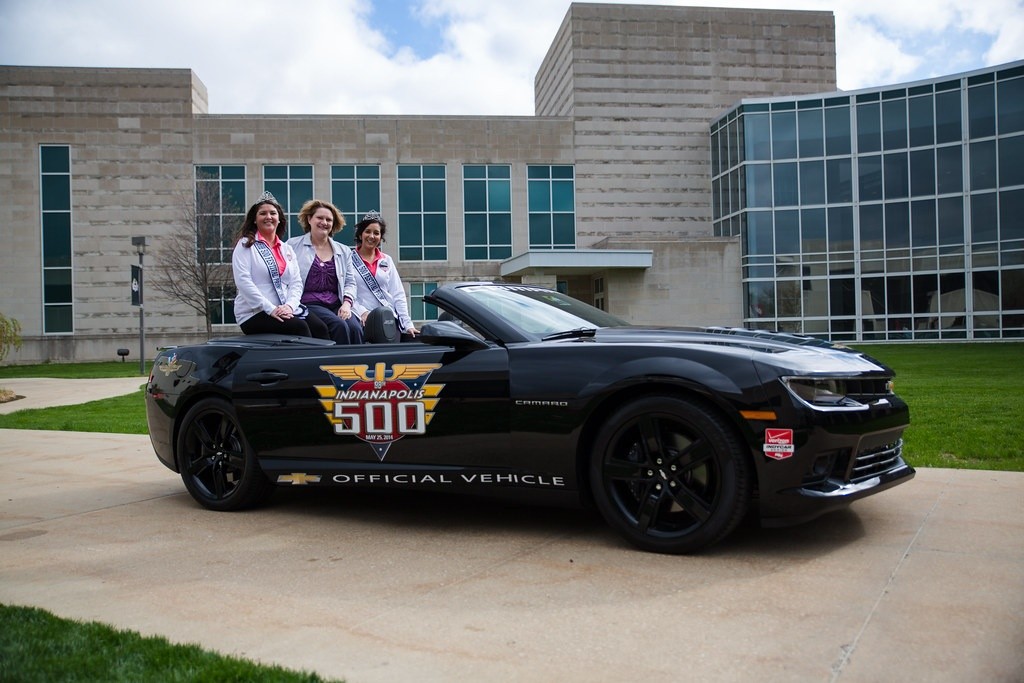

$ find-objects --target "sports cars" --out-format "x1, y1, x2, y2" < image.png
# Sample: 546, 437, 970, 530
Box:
144, 282, 918, 555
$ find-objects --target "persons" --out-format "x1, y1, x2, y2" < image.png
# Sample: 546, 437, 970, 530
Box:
285, 200, 364, 344
351, 210, 421, 343
232, 191, 330, 340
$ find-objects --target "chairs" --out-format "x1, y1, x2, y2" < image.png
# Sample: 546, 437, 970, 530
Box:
439, 311, 463, 328
367, 306, 397, 346
